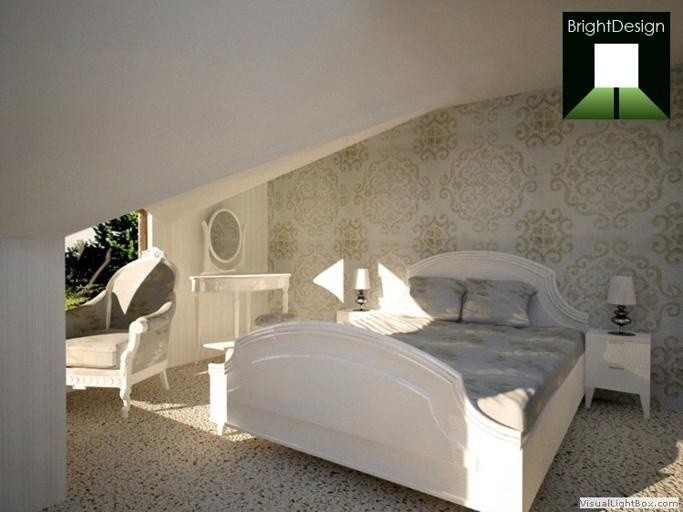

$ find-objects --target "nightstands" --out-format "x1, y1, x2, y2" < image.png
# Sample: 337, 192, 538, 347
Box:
584, 328, 651, 419
336, 309, 373, 324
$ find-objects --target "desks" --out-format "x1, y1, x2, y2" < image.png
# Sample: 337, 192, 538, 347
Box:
190, 273, 291, 366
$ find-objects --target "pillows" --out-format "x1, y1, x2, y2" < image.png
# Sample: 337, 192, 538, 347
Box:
461, 279, 537, 328
400, 277, 465, 321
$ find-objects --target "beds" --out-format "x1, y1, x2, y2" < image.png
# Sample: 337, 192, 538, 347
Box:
208, 250, 590, 512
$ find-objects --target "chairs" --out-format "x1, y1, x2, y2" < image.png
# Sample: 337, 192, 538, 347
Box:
66, 247, 178, 419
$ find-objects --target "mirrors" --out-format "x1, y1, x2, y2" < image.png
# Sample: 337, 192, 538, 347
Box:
202, 208, 247, 275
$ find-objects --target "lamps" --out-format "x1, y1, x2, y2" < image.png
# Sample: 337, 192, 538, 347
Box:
606, 275, 636, 335
352, 269, 370, 311
